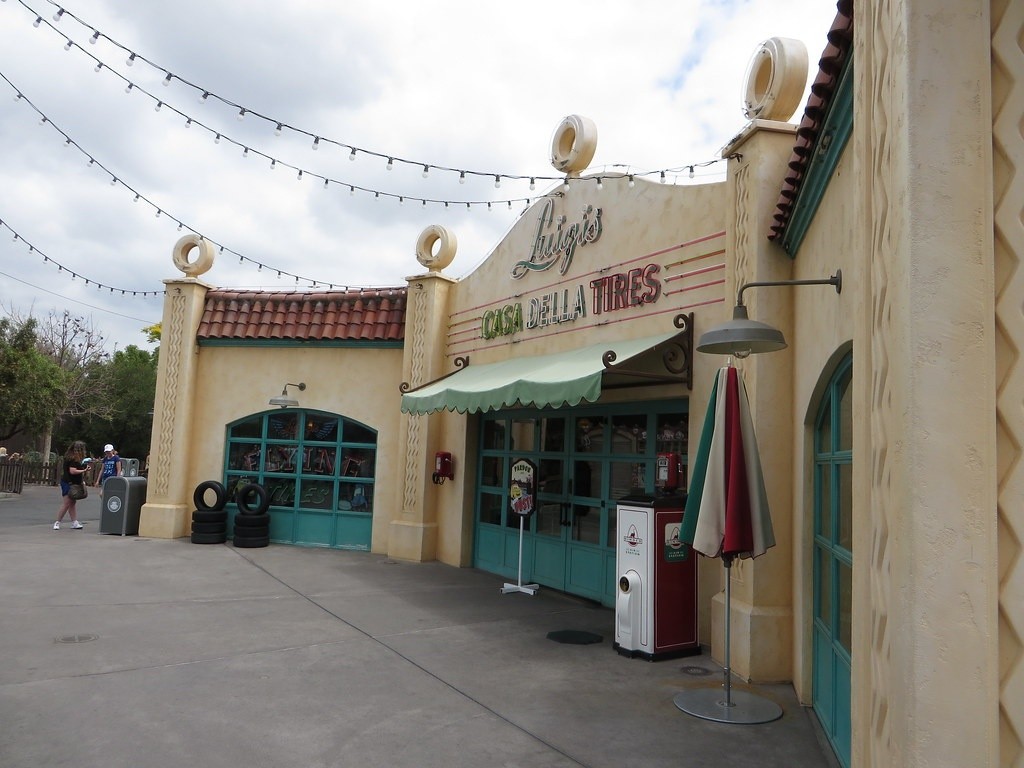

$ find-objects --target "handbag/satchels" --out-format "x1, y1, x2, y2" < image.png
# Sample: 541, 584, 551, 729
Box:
68, 481, 88, 500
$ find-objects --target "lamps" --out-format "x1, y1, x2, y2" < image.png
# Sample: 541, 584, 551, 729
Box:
696, 269, 843, 359
268, 382, 306, 409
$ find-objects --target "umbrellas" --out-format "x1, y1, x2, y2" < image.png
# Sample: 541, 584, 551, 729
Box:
679, 360, 775, 706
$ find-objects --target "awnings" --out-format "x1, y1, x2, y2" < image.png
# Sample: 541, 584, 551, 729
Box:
398, 314, 694, 417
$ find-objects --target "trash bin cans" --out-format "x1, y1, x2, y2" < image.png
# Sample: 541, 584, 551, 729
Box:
120, 459, 140, 476
99, 476, 147, 536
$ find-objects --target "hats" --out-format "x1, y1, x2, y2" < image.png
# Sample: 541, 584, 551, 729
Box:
104, 443, 114, 452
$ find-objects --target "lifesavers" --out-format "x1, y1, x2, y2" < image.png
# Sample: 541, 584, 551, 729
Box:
415, 224, 457, 268
741, 37, 808, 123
191, 480, 228, 544
550, 114, 598, 174
173, 234, 215, 272
233, 484, 270, 549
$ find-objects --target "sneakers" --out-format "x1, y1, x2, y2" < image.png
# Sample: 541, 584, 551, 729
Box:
70, 520, 83, 529
53, 520, 60, 530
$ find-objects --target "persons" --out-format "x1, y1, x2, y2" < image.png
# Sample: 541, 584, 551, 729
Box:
54, 440, 91, 529
94, 443, 122, 497
9, 453, 24, 462
